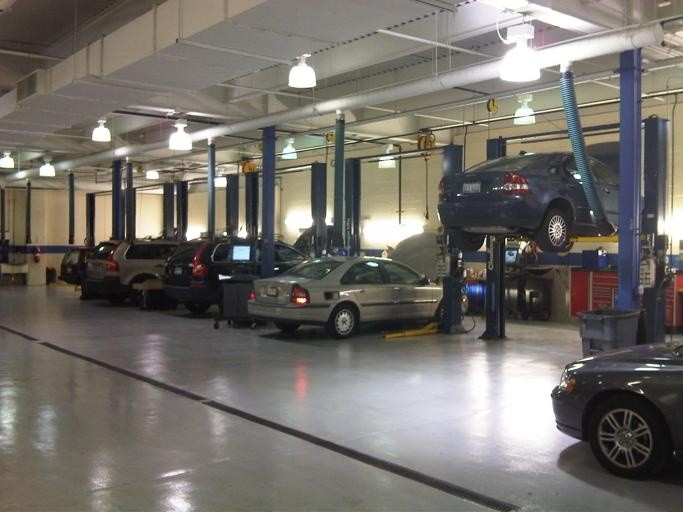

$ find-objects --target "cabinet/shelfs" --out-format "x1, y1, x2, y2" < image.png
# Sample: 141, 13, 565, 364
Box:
569, 271, 683, 333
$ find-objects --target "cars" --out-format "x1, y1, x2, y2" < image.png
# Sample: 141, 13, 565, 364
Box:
436, 152, 621, 251
553, 341, 683, 478
249, 256, 469, 340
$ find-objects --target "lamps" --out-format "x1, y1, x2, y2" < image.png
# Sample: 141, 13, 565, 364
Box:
0, 150, 15, 168
378, 144, 396, 169
39, 152, 56, 177
280, 137, 298, 160
514, 92, 536, 126
495, 9, 542, 84
91, 118, 111, 144
287, 53, 317, 90
168, 119, 193, 153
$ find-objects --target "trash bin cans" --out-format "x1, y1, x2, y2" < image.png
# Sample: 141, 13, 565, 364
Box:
214, 273, 258, 330
580, 308, 640, 356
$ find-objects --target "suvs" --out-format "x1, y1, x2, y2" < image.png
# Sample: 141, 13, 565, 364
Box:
58, 237, 307, 313
